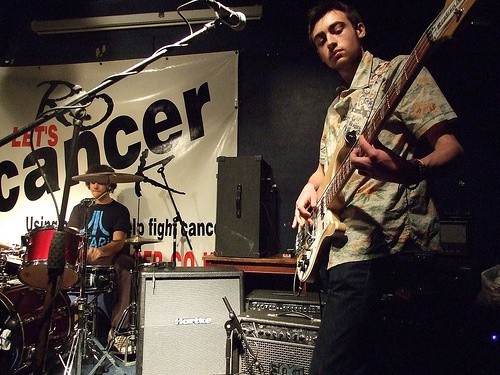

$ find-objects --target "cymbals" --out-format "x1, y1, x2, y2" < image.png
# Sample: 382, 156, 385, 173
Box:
112, 236, 162, 248
72, 171, 145, 183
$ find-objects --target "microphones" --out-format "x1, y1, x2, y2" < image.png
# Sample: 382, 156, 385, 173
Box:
204, 0, 247, 32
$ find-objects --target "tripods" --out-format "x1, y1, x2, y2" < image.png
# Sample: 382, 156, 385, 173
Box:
61, 173, 140, 375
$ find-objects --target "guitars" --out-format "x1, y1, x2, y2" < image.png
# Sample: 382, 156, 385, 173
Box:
295, 0, 474, 283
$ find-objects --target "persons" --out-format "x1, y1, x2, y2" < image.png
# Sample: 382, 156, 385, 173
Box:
292, 2, 467, 375
64, 165, 134, 355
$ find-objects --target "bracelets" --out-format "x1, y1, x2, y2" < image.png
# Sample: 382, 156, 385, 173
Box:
406, 159, 426, 191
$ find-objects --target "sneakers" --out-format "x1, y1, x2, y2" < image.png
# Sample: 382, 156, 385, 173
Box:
107, 329, 136, 354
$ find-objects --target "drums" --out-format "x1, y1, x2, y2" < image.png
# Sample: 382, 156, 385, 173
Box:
0, 277, 72, 375
66, 263, 116, 296
17, 225, 82, 290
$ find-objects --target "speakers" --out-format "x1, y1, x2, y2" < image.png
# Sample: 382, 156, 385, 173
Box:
216, 155, 277, 259
138, 266, 322, 375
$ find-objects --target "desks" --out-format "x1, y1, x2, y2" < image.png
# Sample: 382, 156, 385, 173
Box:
201, 253, 307, 289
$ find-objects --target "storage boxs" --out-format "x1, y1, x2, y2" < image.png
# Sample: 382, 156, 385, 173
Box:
137, 269, 243, 375
